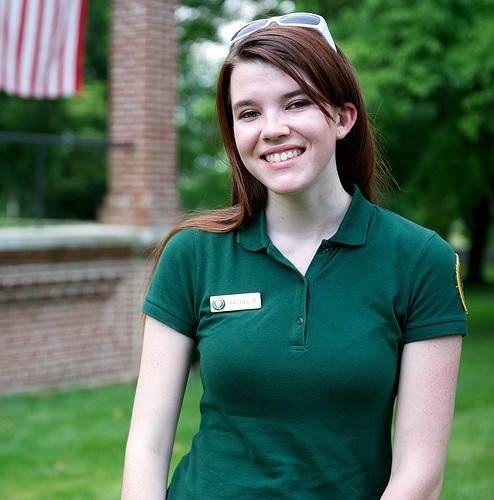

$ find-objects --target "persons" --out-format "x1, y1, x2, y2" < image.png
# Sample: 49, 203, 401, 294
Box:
118, 10, 470, 500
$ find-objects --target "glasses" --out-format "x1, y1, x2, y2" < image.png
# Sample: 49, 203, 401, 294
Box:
230, 12, 338, 54
